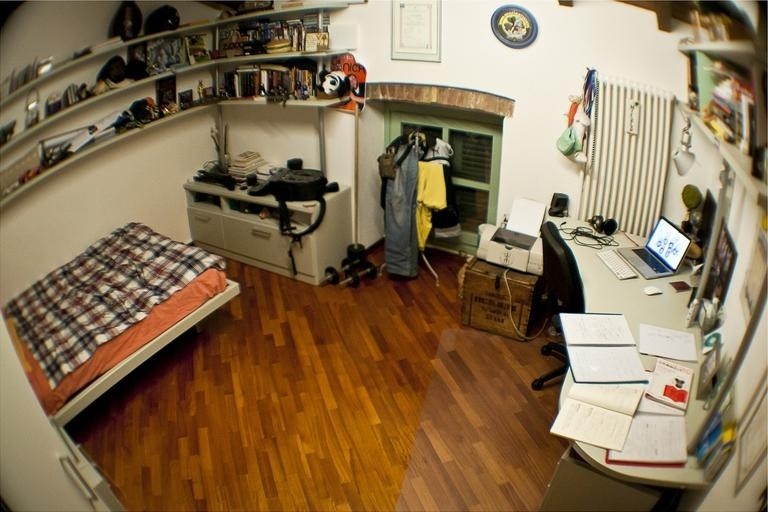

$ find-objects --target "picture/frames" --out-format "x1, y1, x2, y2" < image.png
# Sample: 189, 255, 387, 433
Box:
695, 346, 720, 400
390, 0, 442, 64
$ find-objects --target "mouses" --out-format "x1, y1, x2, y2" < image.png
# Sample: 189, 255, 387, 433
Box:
645, 287, 663, 296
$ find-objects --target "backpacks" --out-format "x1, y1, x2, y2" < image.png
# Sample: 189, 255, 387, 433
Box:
269, 168, 327, 276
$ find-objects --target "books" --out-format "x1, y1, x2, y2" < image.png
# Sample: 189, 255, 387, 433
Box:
700, 59, 757, 151
222, 9, 331, 98
79, 34, 124, 56
155, 74, 177, 117
551, 309, 698, 472
226, 150, 269, 187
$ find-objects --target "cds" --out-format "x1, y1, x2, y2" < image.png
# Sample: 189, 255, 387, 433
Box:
705, 333, 720, 347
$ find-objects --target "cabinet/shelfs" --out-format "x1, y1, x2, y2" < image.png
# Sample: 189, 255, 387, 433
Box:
183, 180, 355, 287
676, 1, 767, 208
0, 0, 349, 214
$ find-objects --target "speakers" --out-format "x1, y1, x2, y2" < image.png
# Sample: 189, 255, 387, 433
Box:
548, 192, 569, 217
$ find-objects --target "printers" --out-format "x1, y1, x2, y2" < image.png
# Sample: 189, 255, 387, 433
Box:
476, 196, 549, 279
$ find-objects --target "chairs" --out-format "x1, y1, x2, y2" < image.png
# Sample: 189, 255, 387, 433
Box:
531, 222, 590, 387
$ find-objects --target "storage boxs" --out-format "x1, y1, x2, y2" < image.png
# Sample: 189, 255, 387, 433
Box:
461, 257, 539, 342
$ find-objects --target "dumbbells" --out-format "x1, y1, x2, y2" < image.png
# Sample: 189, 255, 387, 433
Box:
338, 261, 381, 287
320, 258, 359, 286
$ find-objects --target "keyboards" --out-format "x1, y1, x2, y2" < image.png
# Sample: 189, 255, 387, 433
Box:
596, 248, 640, 283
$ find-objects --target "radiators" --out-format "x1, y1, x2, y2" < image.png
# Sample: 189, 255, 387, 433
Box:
576, 73, 674, 238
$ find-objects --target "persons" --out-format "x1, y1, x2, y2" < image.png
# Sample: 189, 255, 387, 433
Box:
153, 43, 172, 67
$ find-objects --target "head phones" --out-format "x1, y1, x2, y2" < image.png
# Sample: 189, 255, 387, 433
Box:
590, 212, 617, 237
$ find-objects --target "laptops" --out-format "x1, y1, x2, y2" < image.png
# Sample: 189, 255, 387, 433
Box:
617, 213, 692, 281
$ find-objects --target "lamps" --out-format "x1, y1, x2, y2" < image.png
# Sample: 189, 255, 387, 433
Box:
672, 107, 697, 177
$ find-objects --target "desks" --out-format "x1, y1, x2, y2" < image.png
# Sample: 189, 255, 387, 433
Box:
538, 217, 732, 512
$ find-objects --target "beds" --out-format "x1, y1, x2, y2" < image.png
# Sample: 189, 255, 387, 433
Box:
0, 219, 244, 431
